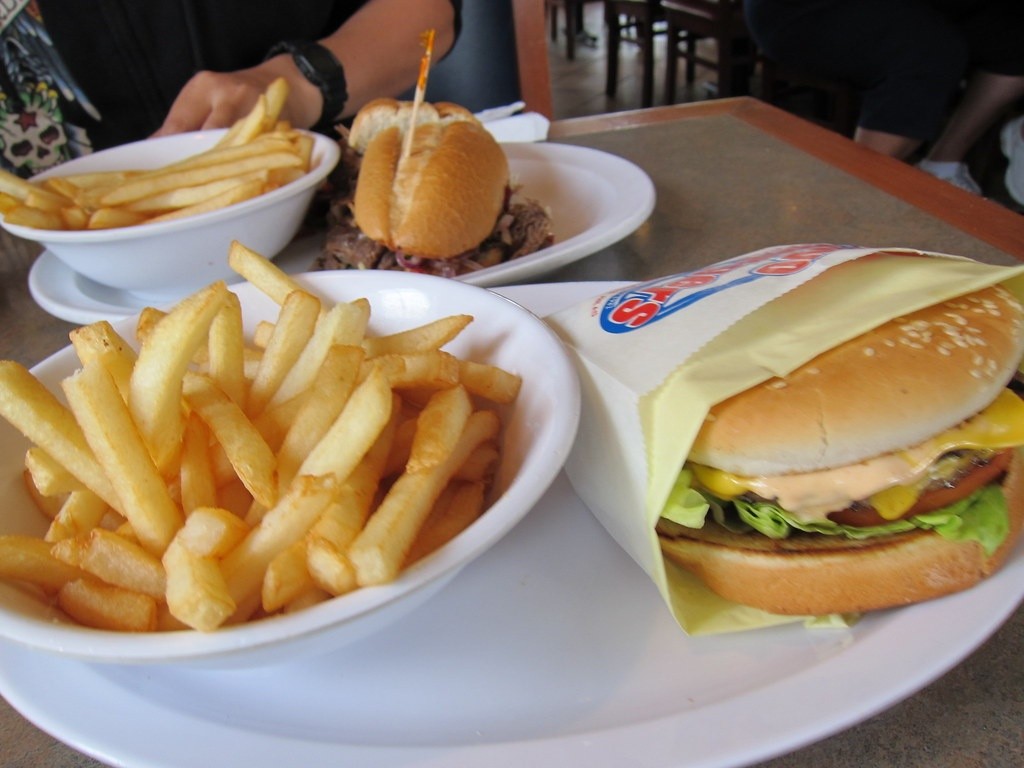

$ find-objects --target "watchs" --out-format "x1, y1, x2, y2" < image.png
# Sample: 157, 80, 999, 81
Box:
262, 38, 349, 131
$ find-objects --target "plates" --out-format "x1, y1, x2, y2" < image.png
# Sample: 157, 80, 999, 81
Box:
28, 143, 658, 287
0, 280, 1023, 768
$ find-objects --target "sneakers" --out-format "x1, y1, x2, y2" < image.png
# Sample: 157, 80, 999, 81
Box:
919, 158, 982, 196
1000, 116, 1024, 205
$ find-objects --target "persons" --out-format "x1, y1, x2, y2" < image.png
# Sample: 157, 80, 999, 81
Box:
743, 0, 1023, 199
0, 0, 463, 180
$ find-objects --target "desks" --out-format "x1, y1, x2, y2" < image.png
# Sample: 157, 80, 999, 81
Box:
0, 97, 1024, 768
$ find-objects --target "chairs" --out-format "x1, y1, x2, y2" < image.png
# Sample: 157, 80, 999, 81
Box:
398, 0, 748, 125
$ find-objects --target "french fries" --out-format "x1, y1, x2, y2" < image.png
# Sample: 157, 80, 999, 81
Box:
0, 241, 523, 632
1, 77, 314, 228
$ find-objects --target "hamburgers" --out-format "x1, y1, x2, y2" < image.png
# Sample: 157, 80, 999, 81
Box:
658, 280, 1024, 615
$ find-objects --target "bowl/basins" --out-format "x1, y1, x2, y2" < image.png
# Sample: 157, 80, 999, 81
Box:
1, 129, 342, 293
0, 268, 582, 662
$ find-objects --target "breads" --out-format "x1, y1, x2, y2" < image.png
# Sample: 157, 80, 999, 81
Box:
347, 98, 509, 257
